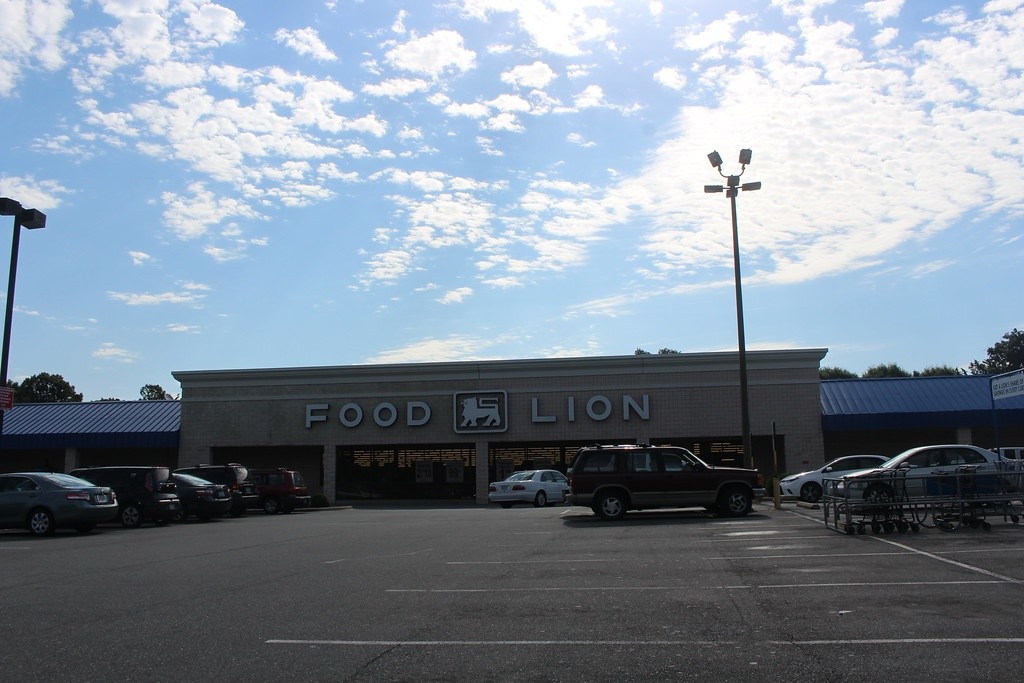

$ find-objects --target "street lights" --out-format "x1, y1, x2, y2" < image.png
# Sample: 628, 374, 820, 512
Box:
704, 149, 761, 469
0, 197, 47, 440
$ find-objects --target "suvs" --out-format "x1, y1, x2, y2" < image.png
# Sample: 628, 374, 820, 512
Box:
68, 466, 181, 527
562, 443, 759, 521
174, 462, 312, 515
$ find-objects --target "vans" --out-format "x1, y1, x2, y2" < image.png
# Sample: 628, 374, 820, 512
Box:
987, 447, 1024, 468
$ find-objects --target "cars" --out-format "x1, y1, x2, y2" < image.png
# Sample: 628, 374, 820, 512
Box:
0, 472, 119, 537
172, 473, 232, 520
779, 455, 891, 502
489, 469, 570, 508
830, 444, 1024, 516
